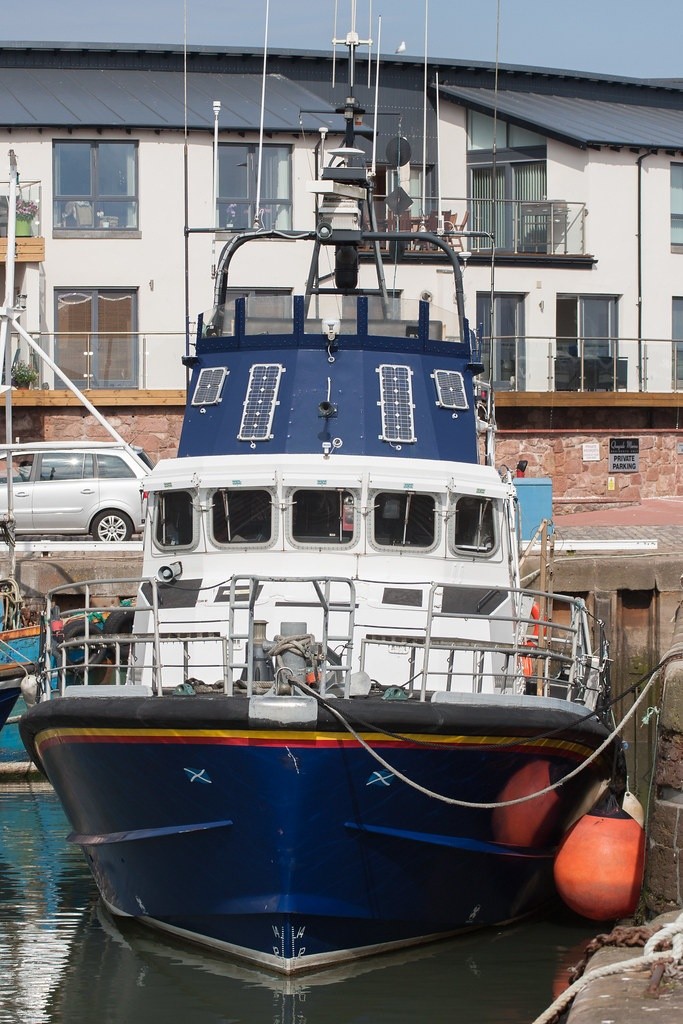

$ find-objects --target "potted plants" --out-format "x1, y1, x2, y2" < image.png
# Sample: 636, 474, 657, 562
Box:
12, 360, 40, 390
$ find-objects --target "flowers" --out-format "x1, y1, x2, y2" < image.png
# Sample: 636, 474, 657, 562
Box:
226, 203, 237, 224
15, 197, 39, 220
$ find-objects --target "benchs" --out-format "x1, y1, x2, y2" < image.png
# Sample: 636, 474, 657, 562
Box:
246, 317, 444, 341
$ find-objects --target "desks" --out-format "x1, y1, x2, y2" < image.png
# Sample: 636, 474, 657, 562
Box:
380, 216, 422, 250
521, 200, 569, 256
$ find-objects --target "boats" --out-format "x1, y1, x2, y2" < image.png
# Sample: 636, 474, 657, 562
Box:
0, 0, 646, 976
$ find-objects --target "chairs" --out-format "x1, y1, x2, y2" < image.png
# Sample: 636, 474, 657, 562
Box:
20, 461, 32, 483
366, 211, 470, 251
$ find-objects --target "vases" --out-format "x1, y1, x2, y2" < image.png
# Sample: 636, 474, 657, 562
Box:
227, 224, 234, 228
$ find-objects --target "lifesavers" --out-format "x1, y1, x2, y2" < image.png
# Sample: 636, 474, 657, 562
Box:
512, 600, 549, 646
51, 604, 135, 671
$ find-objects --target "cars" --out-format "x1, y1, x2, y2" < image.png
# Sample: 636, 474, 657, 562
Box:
0, 440, 154, 541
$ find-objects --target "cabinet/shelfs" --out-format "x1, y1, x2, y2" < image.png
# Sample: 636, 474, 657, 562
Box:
554, 354, 630, 392
